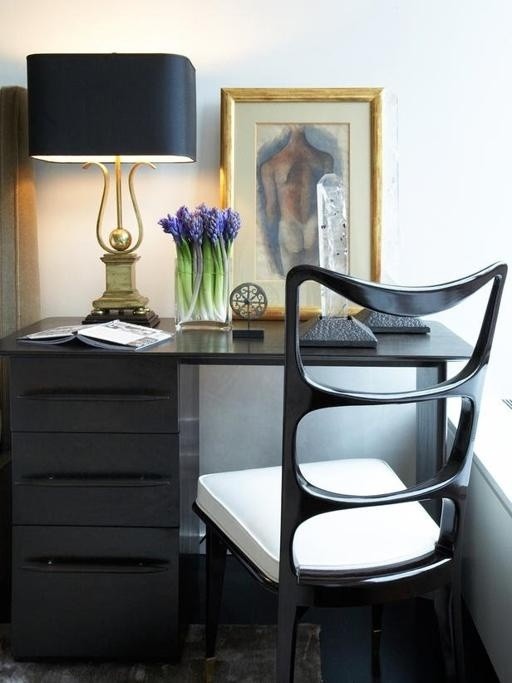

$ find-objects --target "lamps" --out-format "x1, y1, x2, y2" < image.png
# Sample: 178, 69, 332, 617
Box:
25, 52, 197, 329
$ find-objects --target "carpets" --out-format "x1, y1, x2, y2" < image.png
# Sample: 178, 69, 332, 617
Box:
1, 621, 321, 680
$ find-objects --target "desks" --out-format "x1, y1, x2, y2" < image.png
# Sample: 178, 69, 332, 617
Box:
2, 315, 479, 663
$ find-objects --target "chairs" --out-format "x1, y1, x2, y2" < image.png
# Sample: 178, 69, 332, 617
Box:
189, 262, 509, 682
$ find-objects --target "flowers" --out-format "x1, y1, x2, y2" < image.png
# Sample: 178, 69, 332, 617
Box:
157, 200, 241, 322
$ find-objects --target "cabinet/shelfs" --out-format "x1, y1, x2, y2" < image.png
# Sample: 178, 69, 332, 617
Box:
4, 350, 174, 661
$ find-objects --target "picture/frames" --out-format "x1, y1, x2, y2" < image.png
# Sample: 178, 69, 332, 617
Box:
218, 86, 384, 323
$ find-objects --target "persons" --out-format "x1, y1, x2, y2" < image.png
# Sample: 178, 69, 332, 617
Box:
259, 121, 335, 275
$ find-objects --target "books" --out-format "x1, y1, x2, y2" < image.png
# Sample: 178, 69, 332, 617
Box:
15, 318, 175, 352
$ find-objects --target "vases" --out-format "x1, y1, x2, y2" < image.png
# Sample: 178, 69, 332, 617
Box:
173, 257, 232, 333
